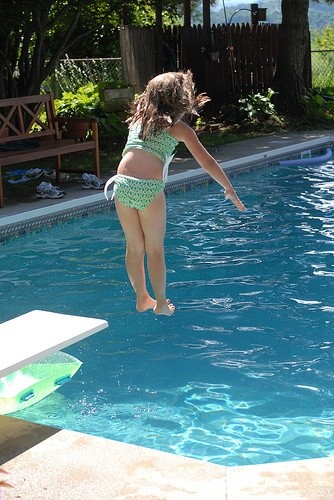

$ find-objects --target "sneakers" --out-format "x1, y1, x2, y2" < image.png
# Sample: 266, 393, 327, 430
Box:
36, 181, 65, 199
80, 172, 106, 189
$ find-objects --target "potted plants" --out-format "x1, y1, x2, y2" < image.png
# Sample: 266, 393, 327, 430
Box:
54, 81, 100, 139
97, 79, 136, 113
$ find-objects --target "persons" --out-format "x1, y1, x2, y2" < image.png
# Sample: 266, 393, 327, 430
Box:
110, 68, 247, 317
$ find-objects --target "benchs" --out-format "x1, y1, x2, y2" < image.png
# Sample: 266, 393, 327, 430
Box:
0, 91, 101, 208
